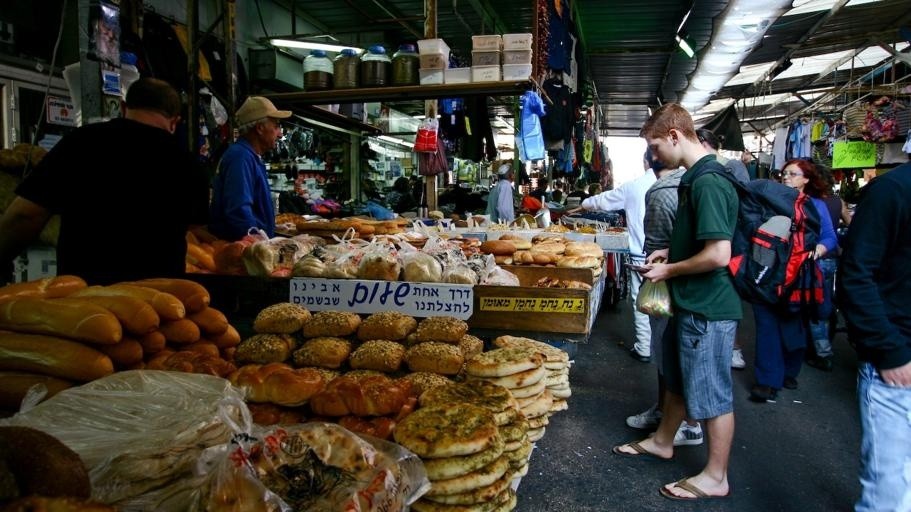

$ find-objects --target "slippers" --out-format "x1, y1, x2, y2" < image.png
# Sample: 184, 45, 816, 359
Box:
660, 475, 732, 499
613, 441, 678, 461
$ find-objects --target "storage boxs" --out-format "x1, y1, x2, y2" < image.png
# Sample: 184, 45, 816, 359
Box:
417, 33, 532, 85
185, 274, 473, 328
461, 228, 630, 251
475, 265, 605, 333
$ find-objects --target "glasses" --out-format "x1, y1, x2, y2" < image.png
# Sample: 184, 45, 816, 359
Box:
783, 172, 802, 177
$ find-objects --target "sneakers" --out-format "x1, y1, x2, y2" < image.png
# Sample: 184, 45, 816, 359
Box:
751, 375, 798, 402
732, 348, 746, 368
627, 403, 663, 428
649, 419, 704, 446
808, 355, 835, 371
630, 346, 650, 363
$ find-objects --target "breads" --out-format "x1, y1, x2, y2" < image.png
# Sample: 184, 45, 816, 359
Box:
184, 232, 254, 274
479, 234, 605, 277
1, 275, 242, 423
228, 303, 486, 441
297, 217, 410, 240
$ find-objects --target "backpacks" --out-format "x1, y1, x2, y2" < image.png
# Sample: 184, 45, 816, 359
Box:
689, 166, 820, 306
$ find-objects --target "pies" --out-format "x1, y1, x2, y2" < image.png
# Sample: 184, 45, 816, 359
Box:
391, 335, 574, 512
0, 369, 405, 511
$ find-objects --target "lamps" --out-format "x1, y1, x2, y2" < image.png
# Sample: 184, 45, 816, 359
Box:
269, 33, 365, 58
676, 32, 698, 58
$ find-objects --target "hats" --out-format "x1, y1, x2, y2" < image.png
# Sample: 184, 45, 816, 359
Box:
125, 78, 181, 114
499, 165, 509, 175
235, 96, 292, 126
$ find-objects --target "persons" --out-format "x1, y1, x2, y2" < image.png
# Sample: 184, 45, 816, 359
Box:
107, 100, 119, 116
0, 77, 214, 288
567, 102, 858, 449
834, 162, 911, 511
383, 165, 601, 225
209, 94, 294, 241
98, 17, 108, 53
105, 28, 120, 56
612, 103, 746, 501
